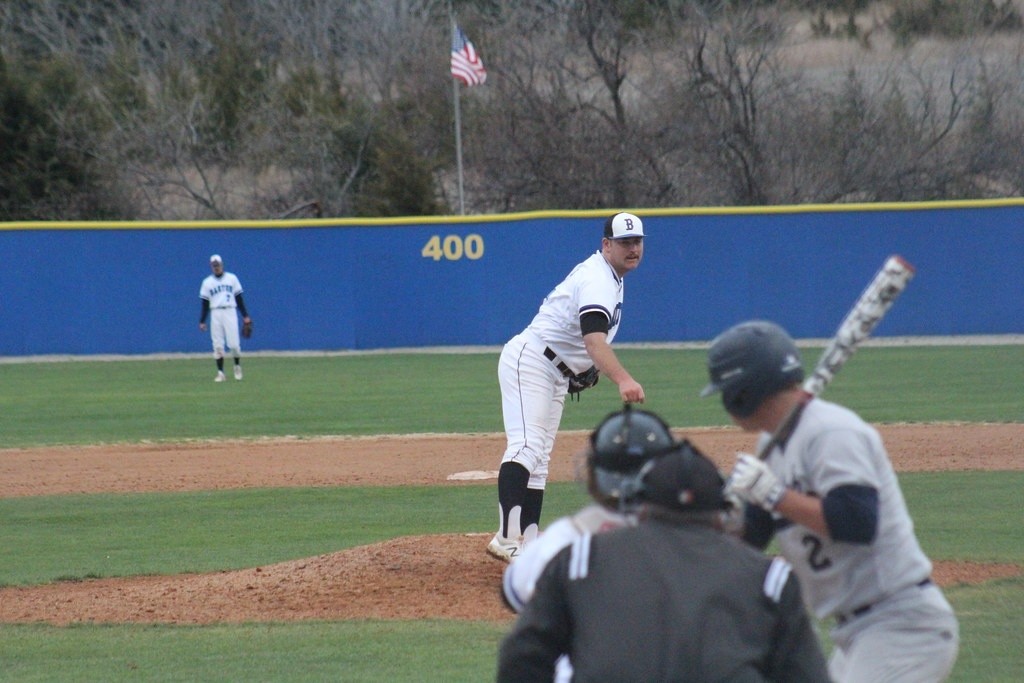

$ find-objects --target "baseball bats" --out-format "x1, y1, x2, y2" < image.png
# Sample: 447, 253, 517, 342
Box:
758, 255, 918, 463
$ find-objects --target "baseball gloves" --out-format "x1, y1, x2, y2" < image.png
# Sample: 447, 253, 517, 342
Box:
568, 365, 599, 393
242, 324, 252, 338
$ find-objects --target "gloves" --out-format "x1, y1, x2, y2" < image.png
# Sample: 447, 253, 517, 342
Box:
728, 453, 785, 512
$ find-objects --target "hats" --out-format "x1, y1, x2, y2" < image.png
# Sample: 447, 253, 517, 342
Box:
622, 440, 734, 512
604, 212, 648, 239
210, 254, 222, 265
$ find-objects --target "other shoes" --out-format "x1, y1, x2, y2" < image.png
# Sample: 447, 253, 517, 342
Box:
214, 371, 225, 382
233, 365, 243, 380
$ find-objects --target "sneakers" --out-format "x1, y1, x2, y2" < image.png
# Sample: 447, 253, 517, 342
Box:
486, 532, 521, 563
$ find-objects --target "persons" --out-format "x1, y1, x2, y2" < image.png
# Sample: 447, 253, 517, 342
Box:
489, 212, 649, 560
701, 320, 960, 683
500, 411, 831, 683
199, 255, 251, 382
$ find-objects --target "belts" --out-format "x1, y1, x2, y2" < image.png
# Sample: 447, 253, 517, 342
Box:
544, 346, 574, 379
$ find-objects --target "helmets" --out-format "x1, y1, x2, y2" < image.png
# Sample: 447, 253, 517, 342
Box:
700, 321, 806, 419
587, 411, 673, 511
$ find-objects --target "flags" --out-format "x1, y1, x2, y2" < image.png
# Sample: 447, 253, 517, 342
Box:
451, 25, 488, 86
